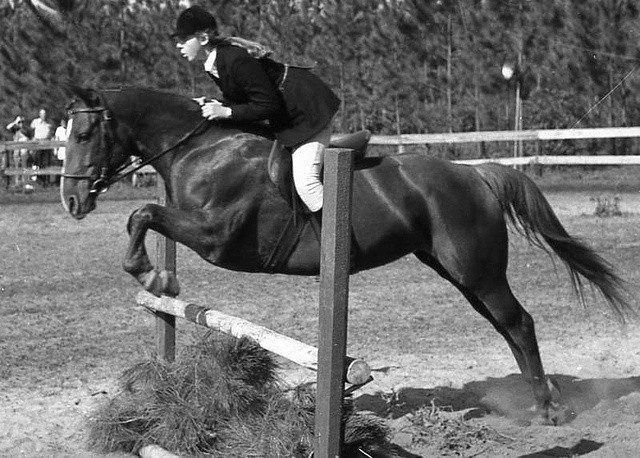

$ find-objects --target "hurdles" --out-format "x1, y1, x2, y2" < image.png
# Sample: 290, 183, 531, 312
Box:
133, 143, 373, 457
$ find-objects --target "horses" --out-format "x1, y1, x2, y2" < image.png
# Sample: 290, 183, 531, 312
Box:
54, 78, 640, 427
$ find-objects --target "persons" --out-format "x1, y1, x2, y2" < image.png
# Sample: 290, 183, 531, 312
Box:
170, 5, 360, 282
5, 107, 68, 187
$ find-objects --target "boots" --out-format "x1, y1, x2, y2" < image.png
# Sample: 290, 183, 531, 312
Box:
314, 207, 361, 282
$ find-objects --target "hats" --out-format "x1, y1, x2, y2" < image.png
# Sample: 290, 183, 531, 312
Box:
171, 6, 216, 36
